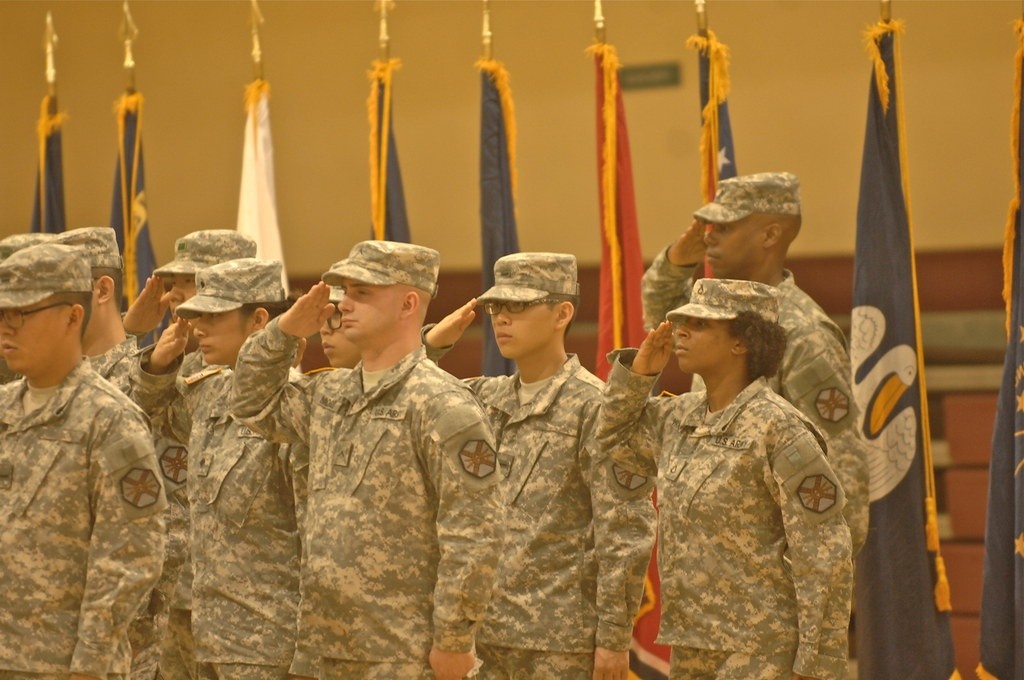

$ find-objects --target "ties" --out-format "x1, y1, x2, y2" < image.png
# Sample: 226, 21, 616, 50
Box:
175, 258, 285, 320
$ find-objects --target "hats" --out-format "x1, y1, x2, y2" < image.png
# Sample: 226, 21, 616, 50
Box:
666, 277, 780, 324
694, 171, 802, 224
0, 227, 124, 308
326, 259, 349, 302
475, 252, 579, 305
152, 229, 256, 275
322, 240, 439, 294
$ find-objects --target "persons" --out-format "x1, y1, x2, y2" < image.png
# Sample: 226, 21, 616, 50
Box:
598, 278, 852, 680
49, 227, 190, 680
120, 229, 256, 680
641, 172, 870, 554
421, 251, 657, 680
0, 231, 57, 384
125, 258, 310, 680
230, 241, 505, 680
293, 285, 360, 373
0, 243, 165, 680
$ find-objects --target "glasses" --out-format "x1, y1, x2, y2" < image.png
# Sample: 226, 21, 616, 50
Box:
0, 301, 73, 329
327, 309, 342, 329
484, 297, 576, 315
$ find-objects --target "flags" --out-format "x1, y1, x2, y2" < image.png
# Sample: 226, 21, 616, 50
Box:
853, 20, 962, 680
977, 17, 1024, 680
586, 44, 672, 680
366, 56, 412, 243
688, 33, 739, 278
32, 94, 68, 234
110, 92, 171, 349
475, 59, 523, 379
239, 81, 302, 376
